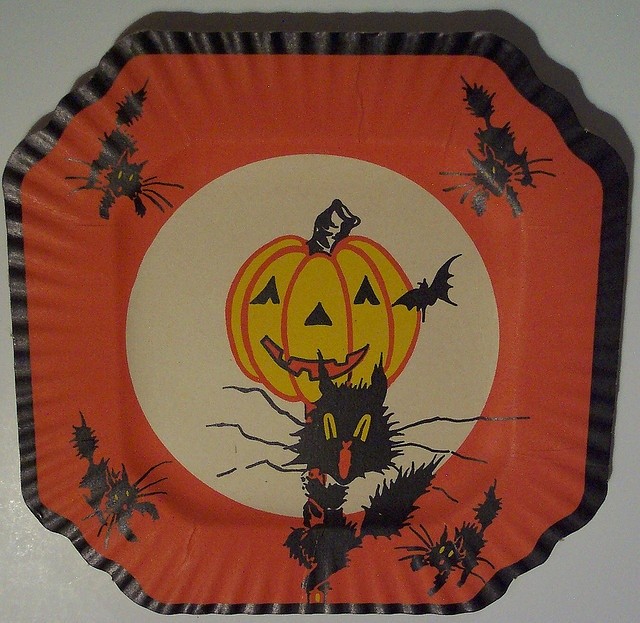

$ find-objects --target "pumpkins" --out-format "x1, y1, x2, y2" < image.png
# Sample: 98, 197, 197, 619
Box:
225, 199, 421, 402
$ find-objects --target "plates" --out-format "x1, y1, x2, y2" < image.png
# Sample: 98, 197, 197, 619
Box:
4, 25, 630, 616
